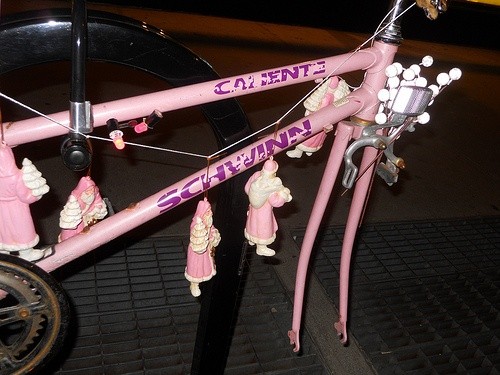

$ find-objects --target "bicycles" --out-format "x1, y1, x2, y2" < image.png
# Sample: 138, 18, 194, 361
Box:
0, 1, 463, 375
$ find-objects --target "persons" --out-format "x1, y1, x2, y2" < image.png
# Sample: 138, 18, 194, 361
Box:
185, 200, 221, 296
287, 76, 340, 158
0, 140, 44, 261
58, 177, 107, 243
245, 160, 292, 256
